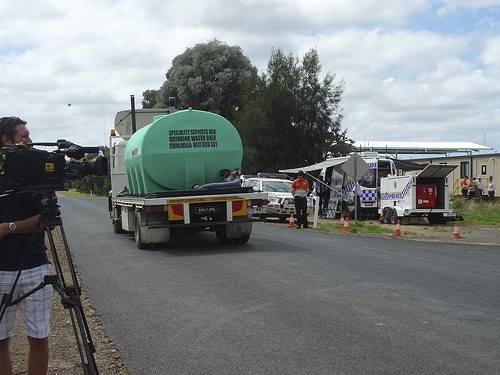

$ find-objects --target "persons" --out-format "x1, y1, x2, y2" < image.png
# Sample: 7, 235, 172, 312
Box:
315, 176, 328, 212
0, 116, 54, 375
461, 176, 483, 202
487, 176, 495, 202
291, 170, 309, 229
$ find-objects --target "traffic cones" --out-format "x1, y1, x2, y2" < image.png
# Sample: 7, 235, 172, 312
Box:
452, 222, 463, 238
340, 217, 350, 233
287, 214, 297, 228
391, 219, 403, 236
338, 214, 345, 226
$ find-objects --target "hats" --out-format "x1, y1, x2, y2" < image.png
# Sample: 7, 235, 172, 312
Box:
298, 170, 304, 176
478, 176, 481, 178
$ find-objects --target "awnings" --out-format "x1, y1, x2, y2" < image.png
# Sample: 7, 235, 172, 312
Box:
279, 159, 348, 198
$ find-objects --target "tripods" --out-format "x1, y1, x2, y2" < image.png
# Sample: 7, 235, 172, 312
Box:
0, 189, 100, 375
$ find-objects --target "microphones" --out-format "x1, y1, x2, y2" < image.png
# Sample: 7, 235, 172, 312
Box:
53, 146, 100, 152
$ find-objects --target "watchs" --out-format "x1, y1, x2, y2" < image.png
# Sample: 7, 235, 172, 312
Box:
9, 222, 16, 234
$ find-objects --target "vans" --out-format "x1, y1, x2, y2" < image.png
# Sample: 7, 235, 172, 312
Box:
312, 157, 397, 220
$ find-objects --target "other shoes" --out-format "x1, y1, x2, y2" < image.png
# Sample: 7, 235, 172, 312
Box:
296, 225, 300, 229
303, 226, 308, 228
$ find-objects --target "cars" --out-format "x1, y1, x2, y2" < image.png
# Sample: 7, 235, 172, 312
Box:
239, 172, 311, 222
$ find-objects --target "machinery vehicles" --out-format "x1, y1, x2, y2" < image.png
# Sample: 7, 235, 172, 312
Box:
107, 95, 271, 249
